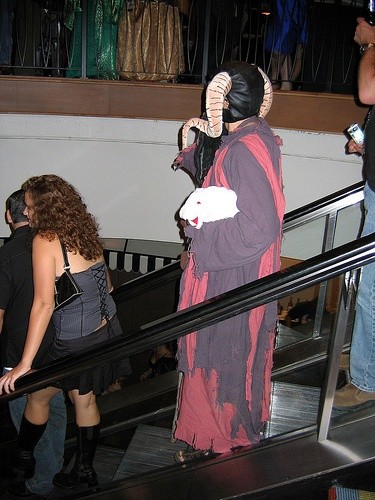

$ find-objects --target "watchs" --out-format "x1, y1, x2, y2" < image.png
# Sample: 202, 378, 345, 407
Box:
360, 42, 375, 54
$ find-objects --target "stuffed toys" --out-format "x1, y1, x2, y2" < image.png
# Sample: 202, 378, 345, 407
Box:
179, 186, 240, 230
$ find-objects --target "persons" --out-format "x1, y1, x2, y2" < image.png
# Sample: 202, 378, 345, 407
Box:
261, 0, 375, 410
0, 0, 368, 92
170, 61, 287, 465
0, 174, 123, 496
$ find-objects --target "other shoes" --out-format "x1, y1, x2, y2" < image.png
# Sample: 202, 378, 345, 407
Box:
332, 382, 375, 412
174, 445, 208, 466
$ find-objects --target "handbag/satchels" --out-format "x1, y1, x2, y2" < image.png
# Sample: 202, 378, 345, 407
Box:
54, 267, 84, 312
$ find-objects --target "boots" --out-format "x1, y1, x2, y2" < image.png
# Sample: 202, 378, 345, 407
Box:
52, 424, 100, 492
0, 414, 50, 495
